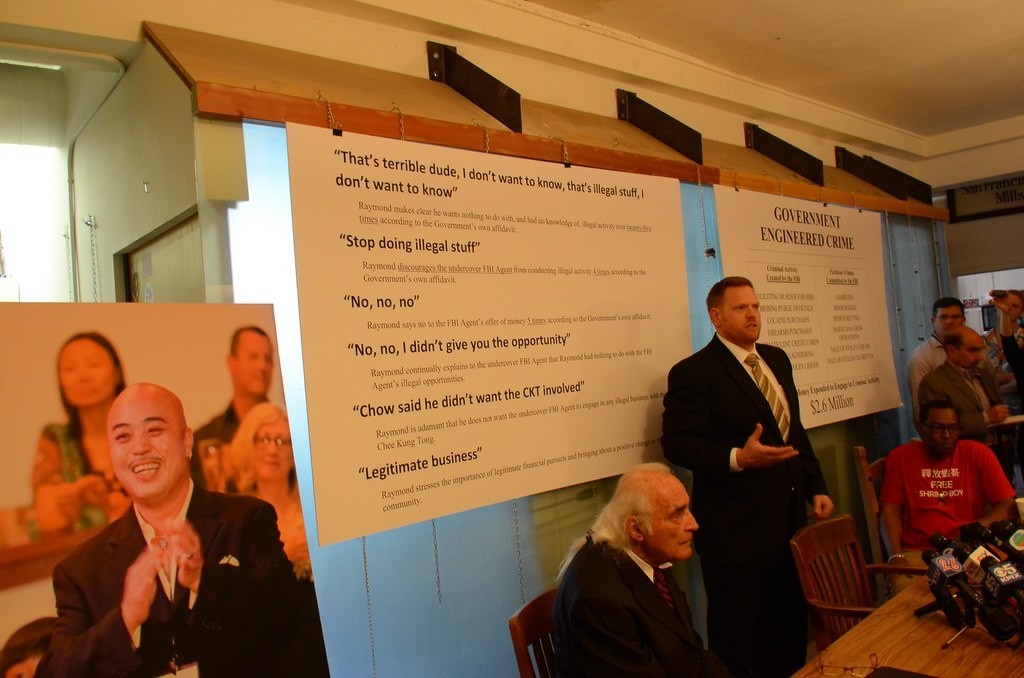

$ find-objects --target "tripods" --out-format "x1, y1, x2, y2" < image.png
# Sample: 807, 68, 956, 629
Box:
940, 596, 990, 650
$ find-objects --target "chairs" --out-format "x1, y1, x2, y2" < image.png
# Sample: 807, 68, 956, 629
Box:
851, 445, 889, 605
790, 514, 929, 652
508, 588, 558, 678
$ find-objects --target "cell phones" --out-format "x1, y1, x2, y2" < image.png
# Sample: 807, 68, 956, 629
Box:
992, 289, 1006, 296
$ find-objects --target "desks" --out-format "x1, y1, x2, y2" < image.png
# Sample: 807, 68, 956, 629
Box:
787, 575, 1024, 678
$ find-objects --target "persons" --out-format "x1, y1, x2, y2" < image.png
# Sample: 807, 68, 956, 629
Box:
907, 291, 1024, 489
879, 399, 1017, 595
33, 326, 310, 678
552, 462, 732, 678
658, 277, 835, 678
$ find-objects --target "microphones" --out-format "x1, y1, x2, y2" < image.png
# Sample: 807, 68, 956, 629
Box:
921, 519, 1024, 642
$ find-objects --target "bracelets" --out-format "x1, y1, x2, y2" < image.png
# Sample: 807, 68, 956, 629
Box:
888, 554, 905, 564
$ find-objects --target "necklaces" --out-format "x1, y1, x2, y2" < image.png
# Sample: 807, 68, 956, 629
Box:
925, 444, 954, 500
152, 534, 172, 550
963, 378, 983, 404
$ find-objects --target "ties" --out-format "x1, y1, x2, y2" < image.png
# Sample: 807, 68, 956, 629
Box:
653, 567, 673, 609
744, 353, 790, 444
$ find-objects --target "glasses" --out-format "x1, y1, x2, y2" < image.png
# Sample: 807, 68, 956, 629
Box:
817, 645, 878, 677
923, 422, 960, 436
254, 436, 290, 448
